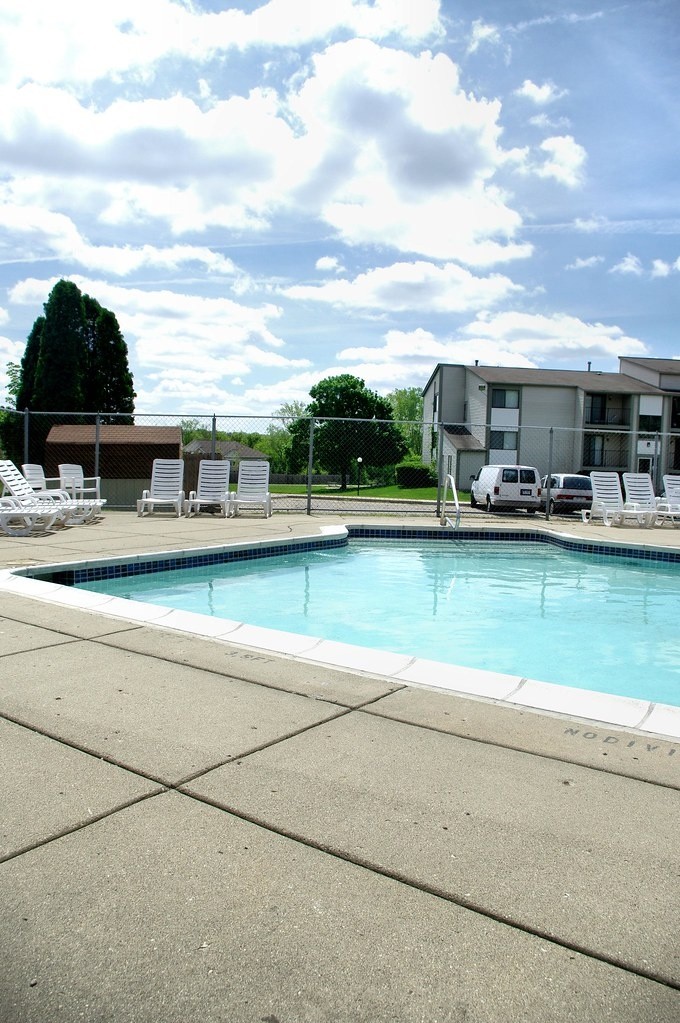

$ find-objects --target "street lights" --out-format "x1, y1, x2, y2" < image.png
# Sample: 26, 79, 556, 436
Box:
357, 457, 362, 496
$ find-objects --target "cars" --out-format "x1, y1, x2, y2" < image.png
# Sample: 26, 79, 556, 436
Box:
540, 473, 593, 514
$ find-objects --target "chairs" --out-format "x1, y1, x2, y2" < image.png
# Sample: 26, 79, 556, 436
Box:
184, 459, 231, 518
656, 474, 680, 529
580, 471, 648, 527
620, 472, 676, 529
594, 414, 617, 424
228, 461, 271, 519
0, 459, 107, 537
136, 459, 185, 518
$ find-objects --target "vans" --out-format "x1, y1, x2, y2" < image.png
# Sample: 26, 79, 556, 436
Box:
469, 464, 542, 516
